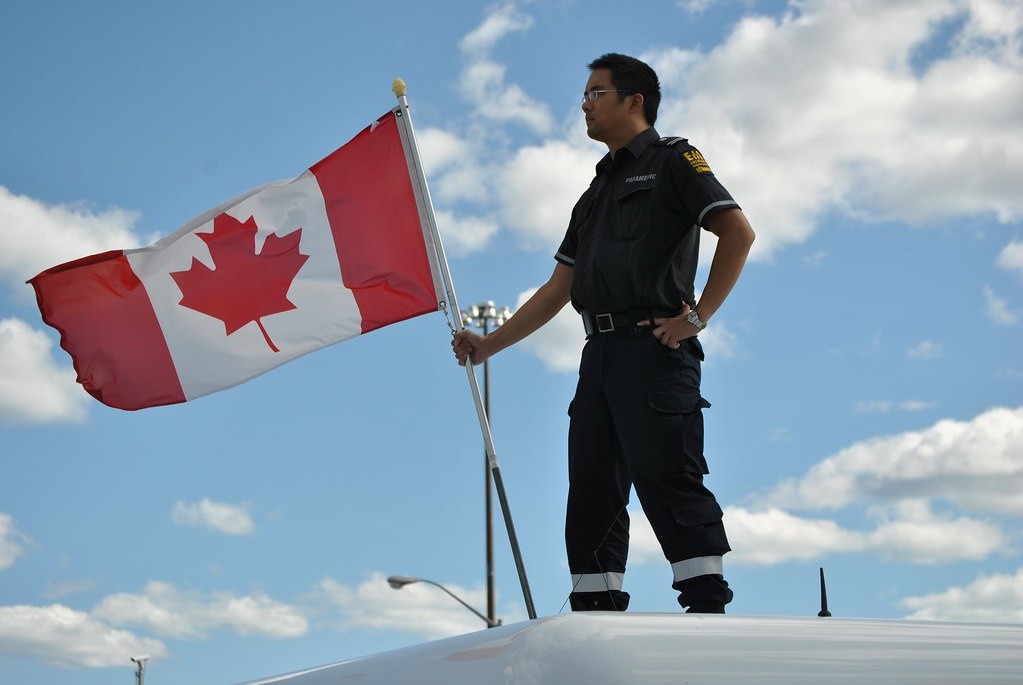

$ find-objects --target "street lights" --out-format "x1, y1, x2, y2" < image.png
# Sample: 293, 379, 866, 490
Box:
459, 301, 514, 631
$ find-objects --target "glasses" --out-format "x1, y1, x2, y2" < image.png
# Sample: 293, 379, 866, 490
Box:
582, 89, 635, 104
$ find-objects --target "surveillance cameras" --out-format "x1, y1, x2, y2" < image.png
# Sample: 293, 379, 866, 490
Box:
131, 654, 150, 662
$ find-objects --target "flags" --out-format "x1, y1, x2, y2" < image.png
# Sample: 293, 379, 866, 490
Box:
22, 104, 450, 415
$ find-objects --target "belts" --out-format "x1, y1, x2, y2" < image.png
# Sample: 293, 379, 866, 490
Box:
585, 312, 682, 336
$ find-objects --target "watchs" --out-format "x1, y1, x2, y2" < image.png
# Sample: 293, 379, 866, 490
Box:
686, 308, 707, 330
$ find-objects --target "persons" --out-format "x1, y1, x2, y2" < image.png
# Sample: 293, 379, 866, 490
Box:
450, 51, 757, 615
